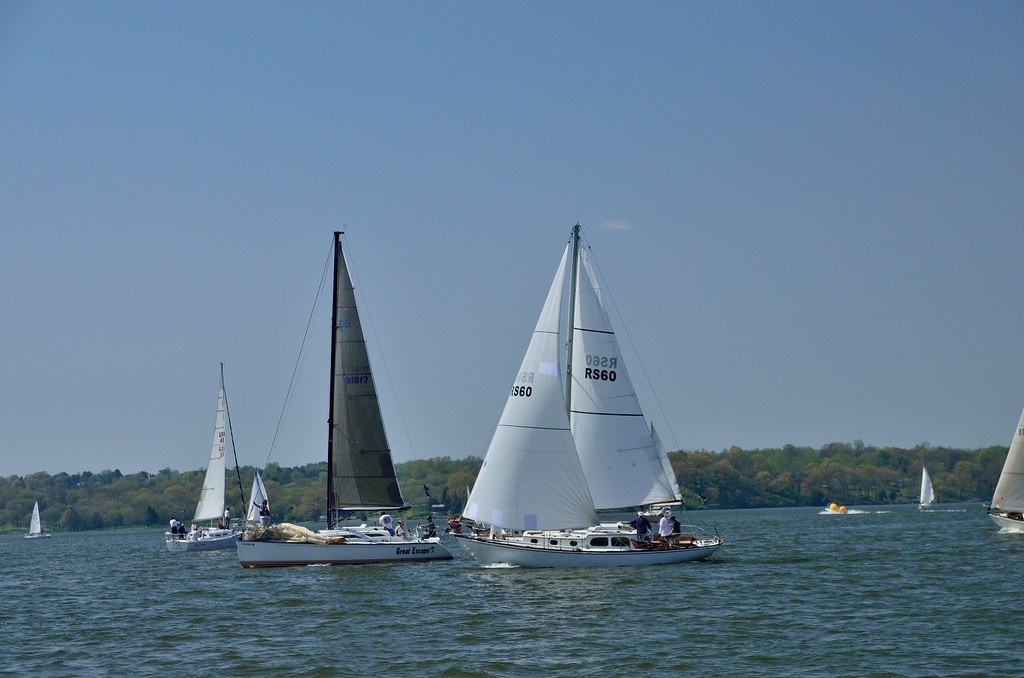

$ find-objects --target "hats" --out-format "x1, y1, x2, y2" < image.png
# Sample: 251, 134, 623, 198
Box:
427, 516, 432, 519
668, 516, 675, 520
181, 524, 184, 526
638, 511, 644, 516
397, 520, 403, 523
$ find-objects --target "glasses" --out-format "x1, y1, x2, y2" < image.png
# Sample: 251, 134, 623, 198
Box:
658, 515, 661, 516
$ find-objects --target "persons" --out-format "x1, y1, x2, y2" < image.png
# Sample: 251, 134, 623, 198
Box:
225, 508, 230, 529
420, 516, 436, 540
395, 520, 406, 535
629, 512, 652, 541
658, 511, 671, 546
177, 524, 188, 539
378, 512, 394, 536
170, 518, 180, 534
253, 499, 271, 528
190, 522, 197, 531
668, 515, 681, 537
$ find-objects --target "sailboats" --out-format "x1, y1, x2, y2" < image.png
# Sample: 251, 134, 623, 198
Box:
234, 232, 454, 567
23, 499, 52, 539
917, 463, 967, 513
449, 222, 725, 567
164, 362, 244, 553
987, 408, 1024, 535
